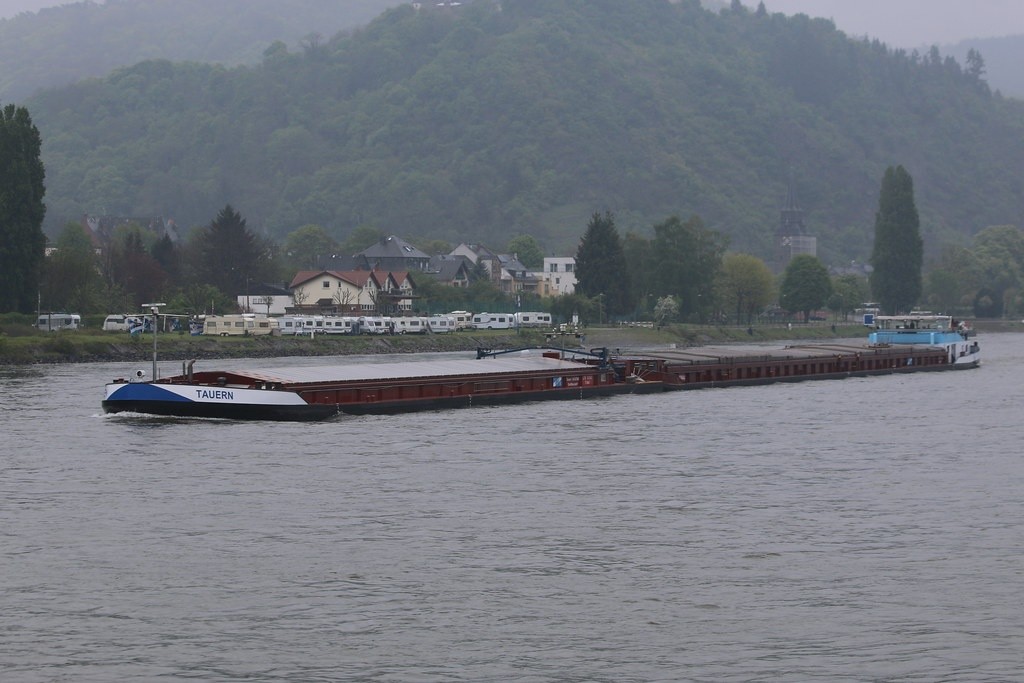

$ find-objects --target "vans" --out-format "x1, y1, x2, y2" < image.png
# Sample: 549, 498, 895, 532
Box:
37, 312, 552, 335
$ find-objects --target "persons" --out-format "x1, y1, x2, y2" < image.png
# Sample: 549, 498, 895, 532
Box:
910, 320, 915, 329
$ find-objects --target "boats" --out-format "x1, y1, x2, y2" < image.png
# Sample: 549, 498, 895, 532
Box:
99, 304, 984, 422
